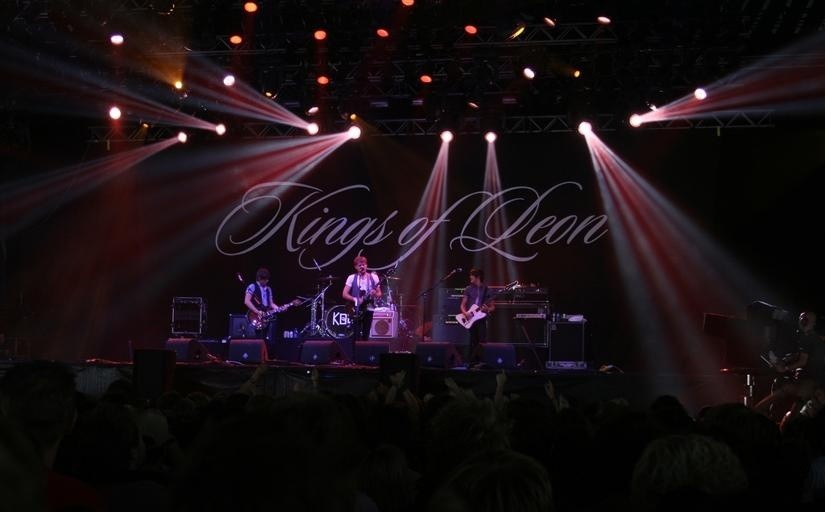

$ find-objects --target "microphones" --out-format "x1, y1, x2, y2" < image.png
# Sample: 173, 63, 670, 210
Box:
236, 271, 243, 282
453, 268, 463, 272
359, 266, 365, 272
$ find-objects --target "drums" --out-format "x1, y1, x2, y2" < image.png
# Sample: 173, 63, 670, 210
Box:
322, 304, 356, 340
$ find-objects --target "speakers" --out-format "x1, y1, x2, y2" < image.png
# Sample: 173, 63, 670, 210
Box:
228, 338, 269, 364
165, 337, 208, 363
300, 340, 349, 366
479, 342, 546, 369
415, 341, 461, 368
354, 340, 390, 365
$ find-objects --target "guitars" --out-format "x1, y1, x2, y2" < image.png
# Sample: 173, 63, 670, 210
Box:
455, 280, 518, 330
344, 266, 394, 318
245, 298, 302, 330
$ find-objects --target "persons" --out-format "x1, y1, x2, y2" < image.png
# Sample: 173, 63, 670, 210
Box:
0, 312, 824, 512
460, 268, 495, 368
244, 267, 288, 360
342, 256, 382, 338
